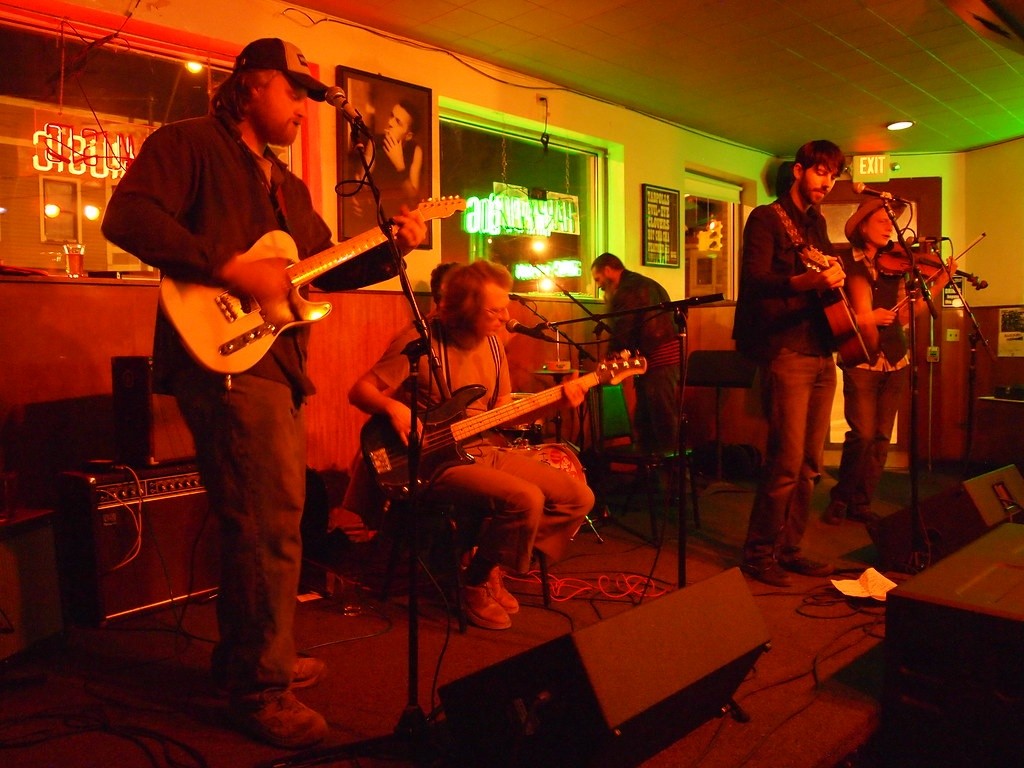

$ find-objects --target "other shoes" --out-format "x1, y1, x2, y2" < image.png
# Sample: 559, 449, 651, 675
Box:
779, 555, 834, 577
745, 559, 794, 587
846, 508, 884, 523
825, 500, 850, 524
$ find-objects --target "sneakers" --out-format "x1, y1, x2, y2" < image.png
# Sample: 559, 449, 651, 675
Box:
226, 693, 328, 749
483, 566, 521, 612
217, 655, 325, 690
447, 579, 512, 630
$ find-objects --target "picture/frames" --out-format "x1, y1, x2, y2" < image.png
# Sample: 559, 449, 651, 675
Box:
641, 180, 682, 269
330, 60, 439, 257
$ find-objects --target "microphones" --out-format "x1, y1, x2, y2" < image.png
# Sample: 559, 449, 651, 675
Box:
325, 87, 374, 138
506, 320, 554, 343
907, 237, 950, 245
851, 182, 908, 205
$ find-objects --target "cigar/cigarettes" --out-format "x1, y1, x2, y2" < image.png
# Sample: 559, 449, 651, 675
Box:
384, 129, 389, 132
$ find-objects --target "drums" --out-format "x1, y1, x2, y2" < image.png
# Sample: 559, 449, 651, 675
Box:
493, 393, 547, 431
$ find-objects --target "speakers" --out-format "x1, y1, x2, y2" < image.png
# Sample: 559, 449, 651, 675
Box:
112, 355, 198, 468
62, 463, 225, 628
0, 508, 65, 673
437, 566, 773, 768
866, 462, 1024, 575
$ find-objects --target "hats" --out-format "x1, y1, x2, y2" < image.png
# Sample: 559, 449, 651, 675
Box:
233, 37, 329, 102
845, 197, 908, 244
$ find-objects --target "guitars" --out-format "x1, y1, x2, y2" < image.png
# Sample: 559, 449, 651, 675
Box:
360, 349, 650, 502
798, 244, 872, 369
158, 195, 467, 377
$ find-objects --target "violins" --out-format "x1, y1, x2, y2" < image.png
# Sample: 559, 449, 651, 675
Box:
875, 240, 988, 290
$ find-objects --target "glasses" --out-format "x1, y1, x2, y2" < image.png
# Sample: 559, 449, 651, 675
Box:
485, 304, 509, 317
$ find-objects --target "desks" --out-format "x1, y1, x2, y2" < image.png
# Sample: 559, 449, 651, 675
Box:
978, 396, 1024, 404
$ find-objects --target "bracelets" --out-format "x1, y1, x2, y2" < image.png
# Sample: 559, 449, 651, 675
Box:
398, 169, 409, 181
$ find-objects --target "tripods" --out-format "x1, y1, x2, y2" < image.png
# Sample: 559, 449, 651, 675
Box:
685, 349, 758, 498
270, 123, 459, 768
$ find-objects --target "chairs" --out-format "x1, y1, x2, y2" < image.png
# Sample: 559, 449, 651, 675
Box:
361, 401, 558, 636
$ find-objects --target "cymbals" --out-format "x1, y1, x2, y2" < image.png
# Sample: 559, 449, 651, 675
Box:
530, 369, 589, 374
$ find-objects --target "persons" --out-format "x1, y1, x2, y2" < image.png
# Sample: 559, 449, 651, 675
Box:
731, 140, 848, 590
100, 37, 426, 752
349, 261, 595, 632
826, 197, 955, 524
591, 252, 680, 449
351, 102, 422, 218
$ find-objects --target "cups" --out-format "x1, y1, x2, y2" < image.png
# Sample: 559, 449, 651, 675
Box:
63, 244, 86, 278
0, 471, 19, 523
341, 576, 364, 616
